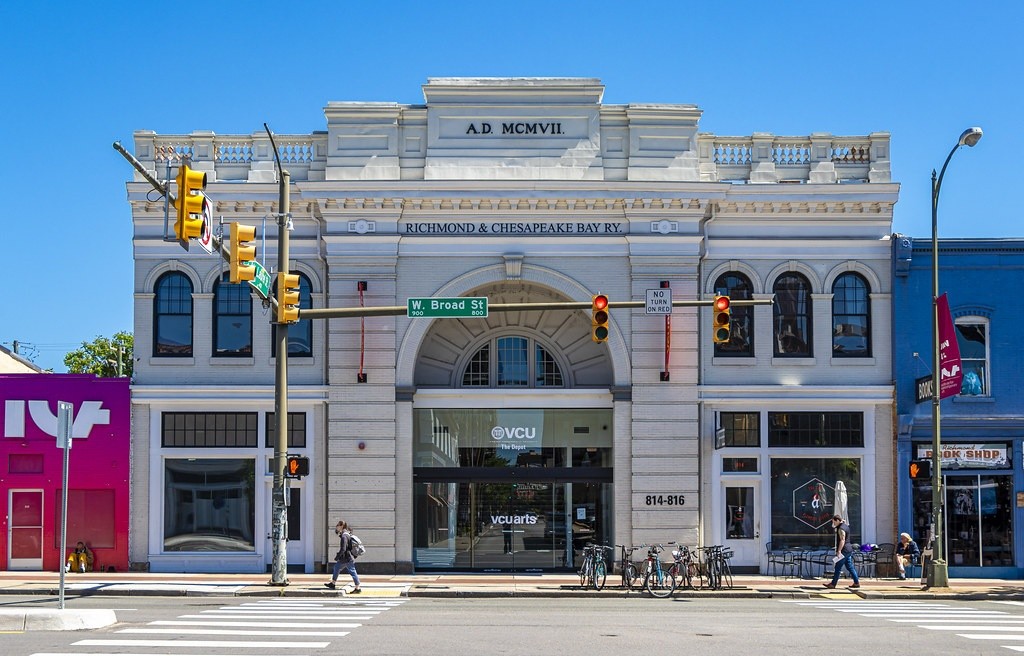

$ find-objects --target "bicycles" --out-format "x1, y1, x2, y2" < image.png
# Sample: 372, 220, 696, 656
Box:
577, 538, 734, 598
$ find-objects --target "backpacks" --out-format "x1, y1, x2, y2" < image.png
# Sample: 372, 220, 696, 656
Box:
343, 531, 366, 557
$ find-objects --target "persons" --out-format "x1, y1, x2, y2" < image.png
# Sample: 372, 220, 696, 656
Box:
501, 511, 514, 556
895, 532, 920, 580
65, 541, 94, 573
324, 520, 365, 595
823, 515, 860, 589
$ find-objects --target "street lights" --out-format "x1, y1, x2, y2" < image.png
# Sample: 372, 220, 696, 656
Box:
925, 126, 984, 587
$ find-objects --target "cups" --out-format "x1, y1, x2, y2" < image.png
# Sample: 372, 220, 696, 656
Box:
101, 564, 103, 571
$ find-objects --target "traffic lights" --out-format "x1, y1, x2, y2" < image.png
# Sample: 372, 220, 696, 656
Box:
229, 221, 257, 285
592, 294, 610, 345
173, 164, 208, 244
909, 460, 931, 480
285, 453, 310, 481
713, 295, 731, 344
276, 271, 301, 324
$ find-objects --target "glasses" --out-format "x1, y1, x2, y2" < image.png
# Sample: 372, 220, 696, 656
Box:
337, 524, 343, 526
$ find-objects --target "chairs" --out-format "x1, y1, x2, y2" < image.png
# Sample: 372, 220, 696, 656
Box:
764, 542, 926, 581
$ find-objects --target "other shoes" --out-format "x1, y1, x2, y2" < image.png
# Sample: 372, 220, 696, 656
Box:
899, 575, 907, 580
503, 552, 509, 555
350, 588, 362, 594
823, 582, 835, 589
848, 583, 860, 588
508, 552, 513, 555
324, 581, 335, 590
